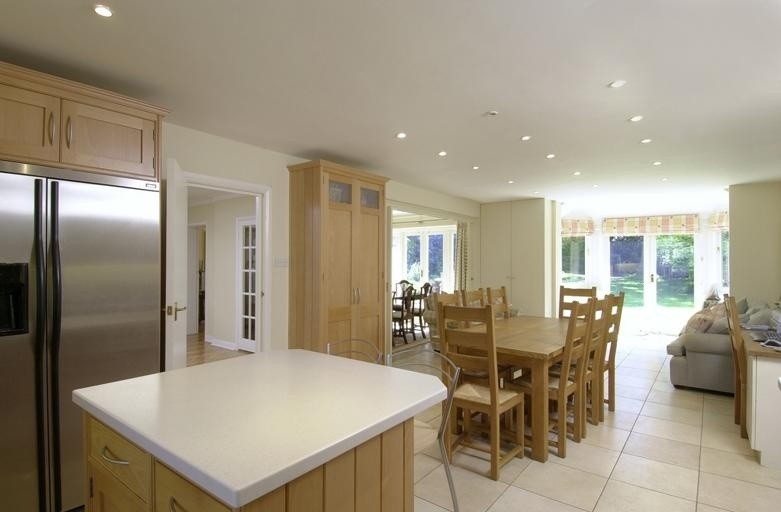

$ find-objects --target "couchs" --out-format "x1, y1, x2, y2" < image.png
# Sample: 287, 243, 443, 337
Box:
666, 301, 738, 396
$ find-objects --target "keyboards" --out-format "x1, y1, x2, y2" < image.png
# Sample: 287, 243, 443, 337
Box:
750, 330, 781, 341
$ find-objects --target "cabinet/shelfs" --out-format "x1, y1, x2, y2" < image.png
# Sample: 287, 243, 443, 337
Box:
288, 158, 392, 366
480, 198, 551, 318
740, 326, 781, 467
0, 59, 173, 184
80, 407, 415, 511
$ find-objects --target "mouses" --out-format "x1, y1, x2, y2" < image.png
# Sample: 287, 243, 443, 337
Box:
765, 339, 781, 346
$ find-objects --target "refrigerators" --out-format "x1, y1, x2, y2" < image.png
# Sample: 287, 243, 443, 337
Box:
0, 159, 165, 511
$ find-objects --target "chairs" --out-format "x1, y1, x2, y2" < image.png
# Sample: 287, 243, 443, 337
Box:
723, 294, 747, 439
392, 279, 433, 347
327, 338, 461, 512
423, 284, 624, 481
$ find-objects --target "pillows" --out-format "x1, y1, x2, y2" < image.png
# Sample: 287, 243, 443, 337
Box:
685, 306, 730, 335
